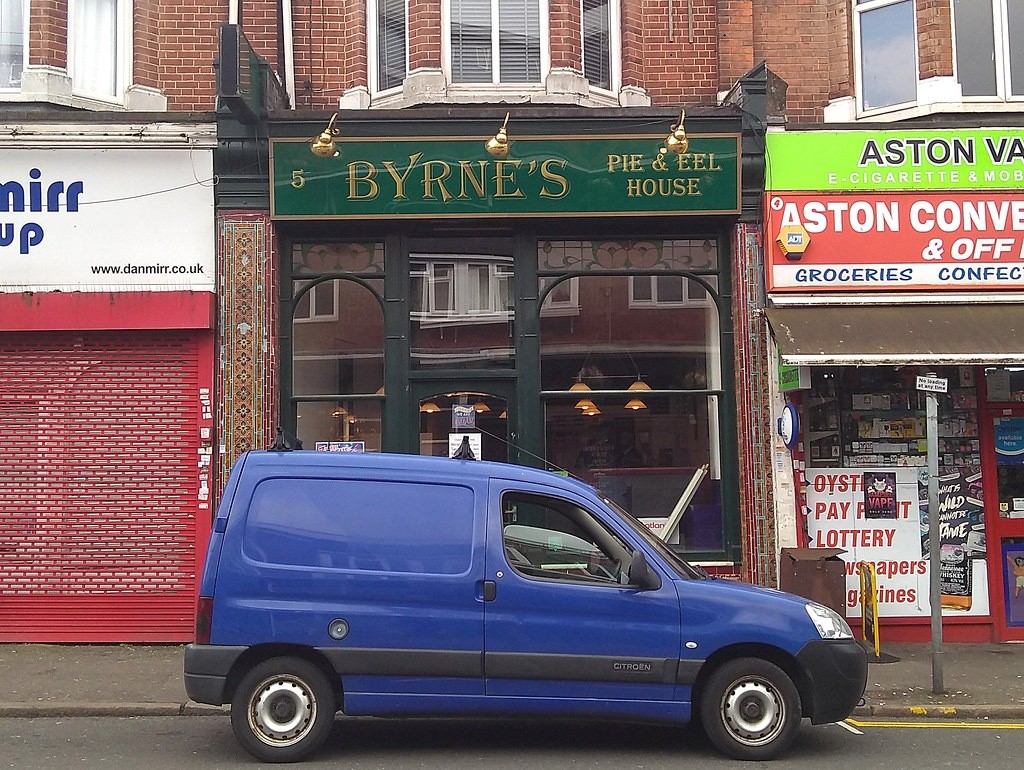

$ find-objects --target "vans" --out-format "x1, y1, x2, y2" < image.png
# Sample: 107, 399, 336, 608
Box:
182, 424, 869, 762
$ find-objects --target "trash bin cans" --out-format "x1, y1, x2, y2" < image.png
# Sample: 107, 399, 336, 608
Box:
780, 547, 848, 620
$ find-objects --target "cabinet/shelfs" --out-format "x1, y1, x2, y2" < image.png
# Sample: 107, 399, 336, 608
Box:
808, 368, 981, 468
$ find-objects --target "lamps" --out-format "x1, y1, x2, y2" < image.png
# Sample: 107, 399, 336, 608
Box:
629, 374, 652, 391
421, 401, 441, 413
624, 395, 648, 411
573, 397, 598, 410
310, 113, 340, 157
569, 375, 592, 391
475, 395, 491, 413
485, 113, 512, 157
582, 408, 602, 416
664, 108, 688, 154
